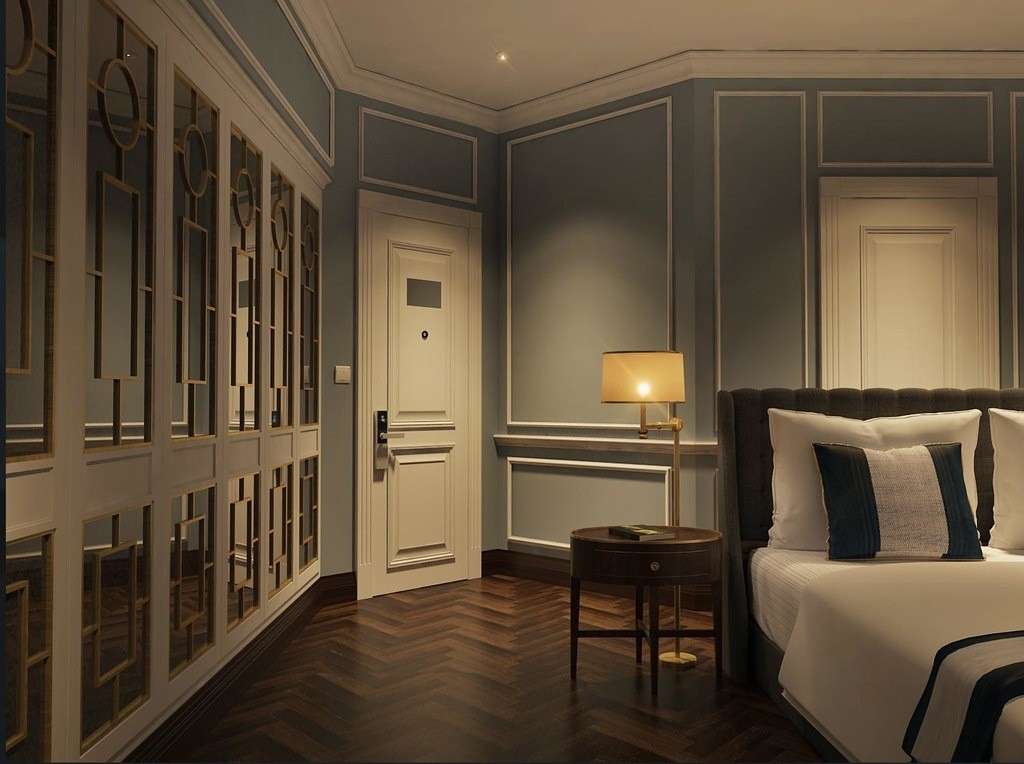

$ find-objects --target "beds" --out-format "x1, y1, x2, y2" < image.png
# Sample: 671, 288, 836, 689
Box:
715, 387, 1024, 764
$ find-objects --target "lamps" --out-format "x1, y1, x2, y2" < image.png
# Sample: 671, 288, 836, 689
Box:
601, 350, 697, 665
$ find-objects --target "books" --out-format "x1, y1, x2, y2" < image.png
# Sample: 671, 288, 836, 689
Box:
608, 525, 675, 541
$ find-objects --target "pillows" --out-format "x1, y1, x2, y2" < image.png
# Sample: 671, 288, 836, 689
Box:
989, 407, 1024, 550
812, 441, 986, 561
767, 407, 982, 550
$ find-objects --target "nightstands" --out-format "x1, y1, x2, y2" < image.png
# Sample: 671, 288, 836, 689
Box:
570, 525, 723, 698
